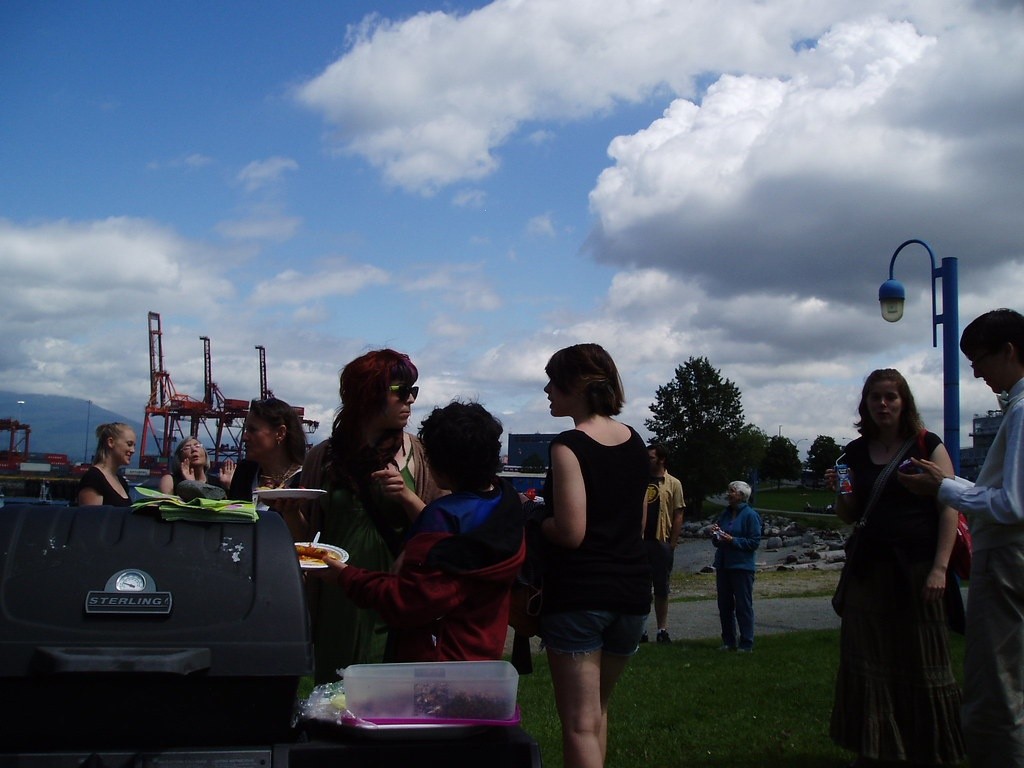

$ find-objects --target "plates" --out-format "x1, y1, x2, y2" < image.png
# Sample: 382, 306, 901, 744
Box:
295, 541, 350, 569
252, 488, 327, 501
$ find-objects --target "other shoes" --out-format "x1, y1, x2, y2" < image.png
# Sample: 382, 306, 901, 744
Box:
744, 647, 753, 653
716, 642, 737, 650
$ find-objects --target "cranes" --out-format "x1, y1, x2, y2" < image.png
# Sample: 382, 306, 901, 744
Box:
139, 309, 320, 473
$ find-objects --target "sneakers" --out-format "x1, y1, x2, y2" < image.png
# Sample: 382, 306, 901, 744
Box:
639, 634, 649, 643
656, 629, 672, 644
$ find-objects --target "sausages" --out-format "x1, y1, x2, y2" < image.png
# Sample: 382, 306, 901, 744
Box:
295, 545, 328, 558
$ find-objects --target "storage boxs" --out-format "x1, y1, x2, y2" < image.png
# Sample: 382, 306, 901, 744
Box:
342, 660, 520, 720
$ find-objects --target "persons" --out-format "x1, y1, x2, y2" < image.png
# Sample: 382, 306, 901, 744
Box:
640, 444, 686, 643
710, 480, 761, 652
518, 343, 650, 768
160, 436, 238, 501
898, 309, 1024, 768
76, 422, 137, 506
262, 349, 453, 688
825, 368, 967, 768
310, 403, 525, 665
228, 398, 309, 521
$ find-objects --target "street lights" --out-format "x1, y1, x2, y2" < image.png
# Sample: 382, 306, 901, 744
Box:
16, 400, 26, 451
84, 399, 93, 463
880, 237, 963, 477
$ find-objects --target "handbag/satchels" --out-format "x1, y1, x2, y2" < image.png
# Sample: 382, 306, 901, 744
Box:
832, 528, 861, 617
918, 430, 973, 581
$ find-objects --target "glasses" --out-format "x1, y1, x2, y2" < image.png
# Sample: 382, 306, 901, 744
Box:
179, 443, 203, 453
971, 337, 1017, 370
388, 385, 418, 400
